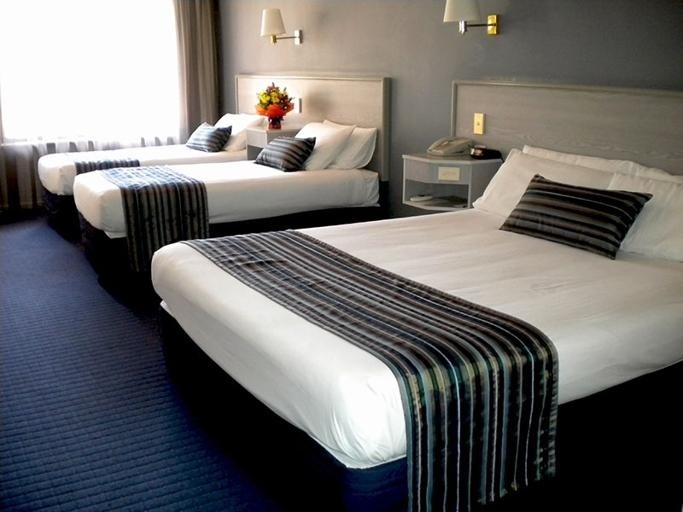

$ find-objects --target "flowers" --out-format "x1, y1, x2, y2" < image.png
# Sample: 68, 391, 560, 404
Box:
254, 81, 294, 117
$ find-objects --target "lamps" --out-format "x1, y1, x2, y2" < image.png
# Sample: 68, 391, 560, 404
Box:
260, 8, 303, 45
442, 0, 500, 36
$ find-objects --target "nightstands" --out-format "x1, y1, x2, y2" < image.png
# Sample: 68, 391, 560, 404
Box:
245, 125, 301, 161
401, 146, 503, 218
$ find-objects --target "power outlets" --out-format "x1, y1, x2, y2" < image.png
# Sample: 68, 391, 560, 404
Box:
292, 96, 302, 114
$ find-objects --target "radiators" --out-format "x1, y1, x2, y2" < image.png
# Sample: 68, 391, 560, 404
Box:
0, 136, 178, 212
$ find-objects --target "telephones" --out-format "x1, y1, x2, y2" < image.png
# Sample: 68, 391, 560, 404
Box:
426, 136, 473, 156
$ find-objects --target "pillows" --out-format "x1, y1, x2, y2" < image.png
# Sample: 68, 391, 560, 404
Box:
471, 141, 616, 253
185, 119, 233, 153
253, 135, 317, 171
295, 121, 356, 172
213, 112, 265, 152
322, 119, 378, 170
498, 172, 654, 261
607, 156, 683, 261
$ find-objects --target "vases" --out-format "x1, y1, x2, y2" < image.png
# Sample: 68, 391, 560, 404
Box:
266, 115, 282, 130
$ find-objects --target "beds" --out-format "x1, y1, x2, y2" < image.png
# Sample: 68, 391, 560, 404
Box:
35, 143, 266, 233
148, 208, 683, 512
72, 160, 387, 288
